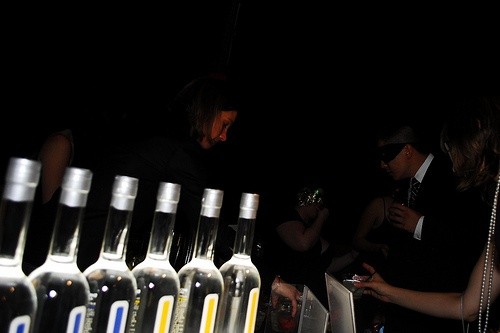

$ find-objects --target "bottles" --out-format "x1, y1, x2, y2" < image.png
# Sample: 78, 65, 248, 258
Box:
131, 182, 180, 333
219, 193, 261, 333
28, 167, 93, 333
83, 175, 138, 333
0, 157, 42, 333
178, 188, 224, 333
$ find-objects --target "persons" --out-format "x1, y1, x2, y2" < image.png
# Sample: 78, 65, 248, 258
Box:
352, 95, 500, 333
0, 76, 265, 266
273, 185, 360, 285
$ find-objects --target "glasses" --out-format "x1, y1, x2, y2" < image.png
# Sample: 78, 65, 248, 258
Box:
320, 194, 328, 201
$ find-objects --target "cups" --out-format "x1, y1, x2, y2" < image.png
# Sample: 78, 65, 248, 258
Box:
277, 296, 301, 333
343, 279, 365, 306
391, 199, 408, 225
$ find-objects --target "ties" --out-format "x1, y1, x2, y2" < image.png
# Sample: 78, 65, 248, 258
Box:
407, 177, 419, 208
